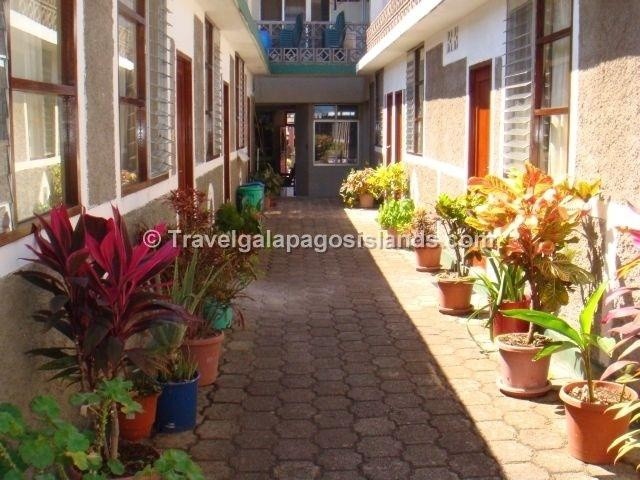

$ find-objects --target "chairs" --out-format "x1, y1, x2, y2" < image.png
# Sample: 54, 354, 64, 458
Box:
279, 11, 346, 63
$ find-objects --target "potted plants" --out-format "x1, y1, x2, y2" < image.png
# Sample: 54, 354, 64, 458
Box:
0, 164, 282, 480
340, 160, 640, 475
315, 135, 348, 164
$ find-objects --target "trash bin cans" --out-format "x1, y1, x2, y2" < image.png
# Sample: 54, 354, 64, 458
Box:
238, 181, 265, 214
259, 26, 271, 53
